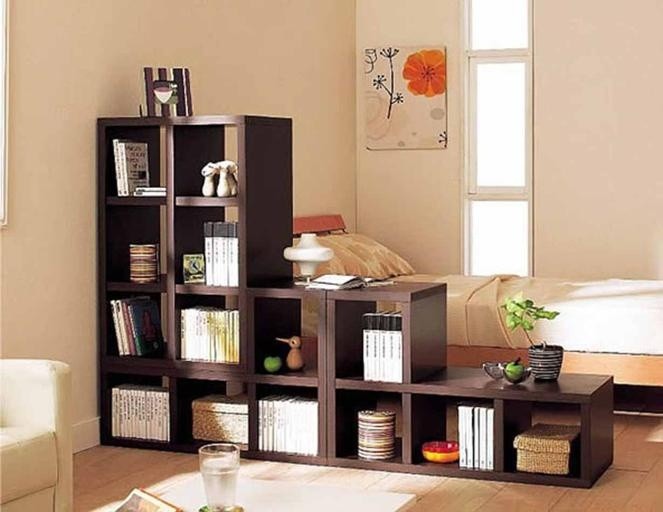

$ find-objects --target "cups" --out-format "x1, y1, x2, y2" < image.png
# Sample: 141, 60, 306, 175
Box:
356, 408, 396, 460
198, 443, 240, 511
129, 243, 158, 284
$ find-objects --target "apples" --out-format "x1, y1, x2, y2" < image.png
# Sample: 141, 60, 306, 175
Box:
264, 356, 281, 373
505, 361, 526, 384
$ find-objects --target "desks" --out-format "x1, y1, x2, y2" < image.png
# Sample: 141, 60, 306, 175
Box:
159, 481, 416, 512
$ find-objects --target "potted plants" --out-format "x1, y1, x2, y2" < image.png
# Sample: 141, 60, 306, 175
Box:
502, 292, 563, 382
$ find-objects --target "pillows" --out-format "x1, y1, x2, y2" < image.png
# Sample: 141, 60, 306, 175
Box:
292, 235, 375, 281
327, 235, 413, 281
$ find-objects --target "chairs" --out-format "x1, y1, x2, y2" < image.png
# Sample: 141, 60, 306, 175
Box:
0, 359, 74, 512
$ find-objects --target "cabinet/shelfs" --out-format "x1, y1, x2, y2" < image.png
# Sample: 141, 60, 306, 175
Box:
97, 116, 613, 489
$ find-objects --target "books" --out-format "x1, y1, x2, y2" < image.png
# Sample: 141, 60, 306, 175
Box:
113, 136, 167, 198
111, 383, 170, 442
111, 295, 163, 356
257, 393, 319, 457
204, 220, 240, 288
362, 310, 403, 384
115, 486, 184, 512
457, 400, 494, 471
179, 305, 240, 365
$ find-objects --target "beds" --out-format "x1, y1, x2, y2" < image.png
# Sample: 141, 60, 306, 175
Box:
293, 215, 663, 387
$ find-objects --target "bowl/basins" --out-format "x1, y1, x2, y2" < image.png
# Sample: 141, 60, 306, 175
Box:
421, 442, 458, 463
501, 365, 532, 385
482, 362, 508, 380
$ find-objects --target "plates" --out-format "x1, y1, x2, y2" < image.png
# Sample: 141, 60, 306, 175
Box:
198, 505, 244, 512
